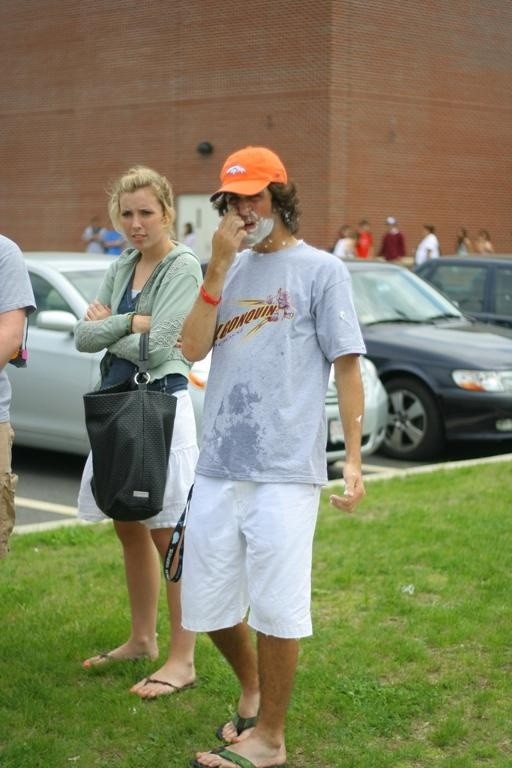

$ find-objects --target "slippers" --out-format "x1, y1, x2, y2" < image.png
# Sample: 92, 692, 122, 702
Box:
217, 712, 258, 744
190, 744, 256, 768
129, 675, 194, 700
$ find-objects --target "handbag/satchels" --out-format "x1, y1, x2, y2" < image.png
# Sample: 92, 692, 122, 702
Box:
84, 380, 177, 521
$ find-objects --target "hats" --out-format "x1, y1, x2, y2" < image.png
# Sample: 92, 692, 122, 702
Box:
210, 146, 288, 203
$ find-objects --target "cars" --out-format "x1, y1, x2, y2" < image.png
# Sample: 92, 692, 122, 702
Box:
412, 255, 512, 331
3, 249, 389, 472
331, 255, 511, 462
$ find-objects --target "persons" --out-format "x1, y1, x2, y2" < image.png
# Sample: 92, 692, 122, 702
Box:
67, 168, 205, 700
0, 233, 37, 568
375, 216, 495, 272
177, 141, 371, 767
101, 217, 127, 256
351, 220, 374, 260
182, 221, 198, 255
80, 214, 108, 254
331, 223, 355, 258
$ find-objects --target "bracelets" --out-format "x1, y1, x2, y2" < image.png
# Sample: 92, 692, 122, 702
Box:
200, 283, 222, 305
